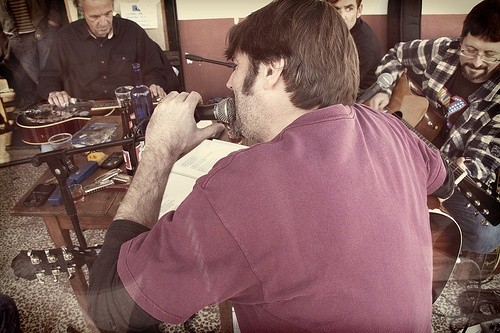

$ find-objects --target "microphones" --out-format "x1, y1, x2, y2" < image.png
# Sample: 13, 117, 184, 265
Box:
356, 72, 393, 104
194, 97, 236, 123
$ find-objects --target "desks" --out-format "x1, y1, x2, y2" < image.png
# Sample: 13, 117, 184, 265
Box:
9, 116, 238, 333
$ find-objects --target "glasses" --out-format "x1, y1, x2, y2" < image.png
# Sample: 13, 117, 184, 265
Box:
459, 44, 500, 64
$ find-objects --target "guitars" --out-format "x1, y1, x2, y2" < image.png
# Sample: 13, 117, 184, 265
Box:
11, 194, 463, 305
384, 72, 500, 227
15, 100, 159, 145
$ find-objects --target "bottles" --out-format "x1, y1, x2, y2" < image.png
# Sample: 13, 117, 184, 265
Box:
116, 107, 143, 176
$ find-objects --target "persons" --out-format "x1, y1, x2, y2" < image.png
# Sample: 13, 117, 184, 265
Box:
323, 0, 384, 106
37, 0, 183, 109
0, 0, 67, 100
361, 1, 500, 255
86, 1, 456, 333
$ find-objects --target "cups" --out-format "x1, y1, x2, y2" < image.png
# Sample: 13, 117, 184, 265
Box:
47, 131, 75, 160
115, 85, 136, 122
69, 184, 85, 204
225, 126, 242, 144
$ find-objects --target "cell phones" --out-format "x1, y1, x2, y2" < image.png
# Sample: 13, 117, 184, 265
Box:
100, 152, 124, 169
24, 184, 56, 206
